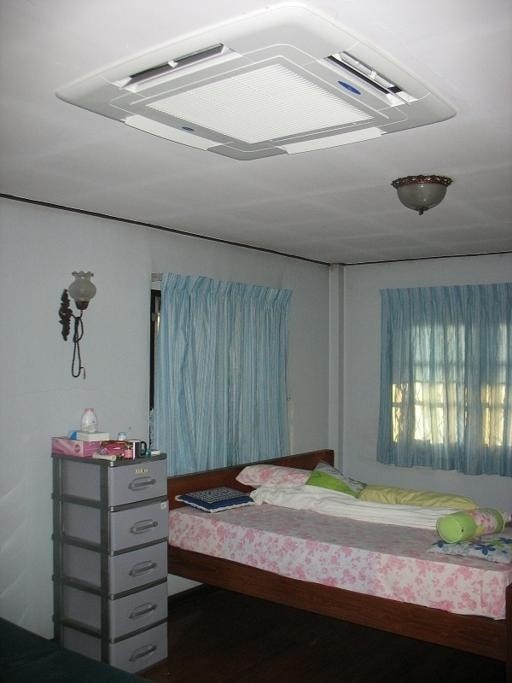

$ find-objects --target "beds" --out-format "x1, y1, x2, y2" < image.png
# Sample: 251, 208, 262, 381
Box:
167, 449, 512, 683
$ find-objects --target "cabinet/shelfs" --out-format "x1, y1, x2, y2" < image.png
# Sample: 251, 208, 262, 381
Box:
51, 453, 169, 674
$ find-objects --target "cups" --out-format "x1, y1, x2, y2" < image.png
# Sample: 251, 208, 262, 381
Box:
127, 438, 147, 459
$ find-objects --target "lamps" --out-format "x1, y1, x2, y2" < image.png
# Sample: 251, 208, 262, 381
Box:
59, 271, 96, 379
391, 174, 453, 216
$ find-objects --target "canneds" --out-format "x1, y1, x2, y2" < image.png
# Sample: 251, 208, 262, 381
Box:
124, 446, 133, 461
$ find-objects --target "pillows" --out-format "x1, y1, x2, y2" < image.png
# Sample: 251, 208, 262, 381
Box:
175, 459, 368, 512
427, 507, 512, 566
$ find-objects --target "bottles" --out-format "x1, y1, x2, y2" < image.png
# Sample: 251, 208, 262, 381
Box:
117, 431, 126, 440
80, 407, 98, 433
